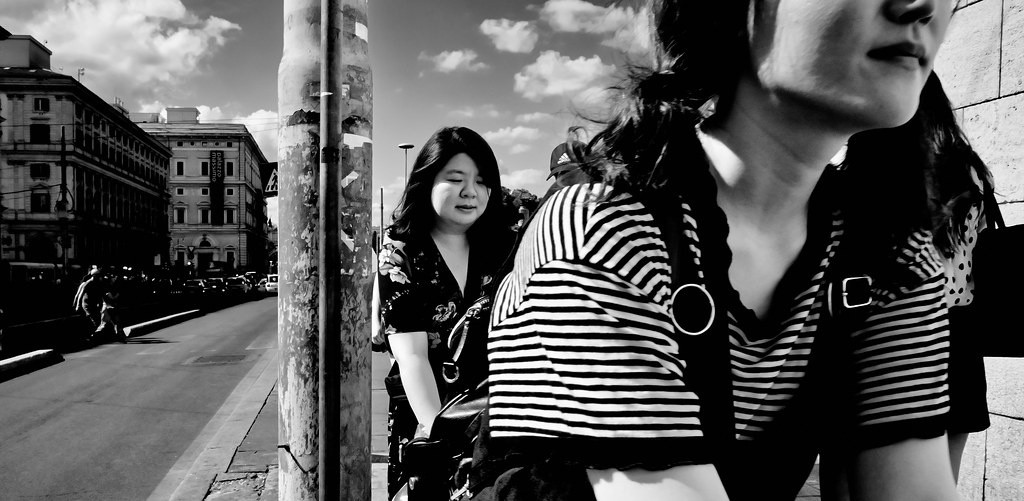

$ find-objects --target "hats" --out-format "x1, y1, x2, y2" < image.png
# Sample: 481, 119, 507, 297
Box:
90, 268, 100, 275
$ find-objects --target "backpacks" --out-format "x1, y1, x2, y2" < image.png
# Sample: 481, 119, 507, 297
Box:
391, 143, 876, 501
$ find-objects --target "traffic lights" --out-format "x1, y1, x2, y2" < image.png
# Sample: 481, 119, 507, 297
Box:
187, 262, 191, 267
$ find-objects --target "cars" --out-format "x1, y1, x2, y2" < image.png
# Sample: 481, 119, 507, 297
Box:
183, 272, 279, 300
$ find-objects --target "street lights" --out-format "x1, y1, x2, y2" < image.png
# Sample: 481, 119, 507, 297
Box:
399, 144, 415, 188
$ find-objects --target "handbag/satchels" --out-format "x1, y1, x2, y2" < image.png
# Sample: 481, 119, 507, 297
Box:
964, 146, 1024, 358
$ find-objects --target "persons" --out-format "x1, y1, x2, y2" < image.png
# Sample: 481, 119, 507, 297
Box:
484, 0, 995, 501
71, 264, 132, 347
372, 127, 520, 501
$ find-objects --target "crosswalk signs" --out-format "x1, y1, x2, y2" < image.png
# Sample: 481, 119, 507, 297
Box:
259, 162, 278, 197
